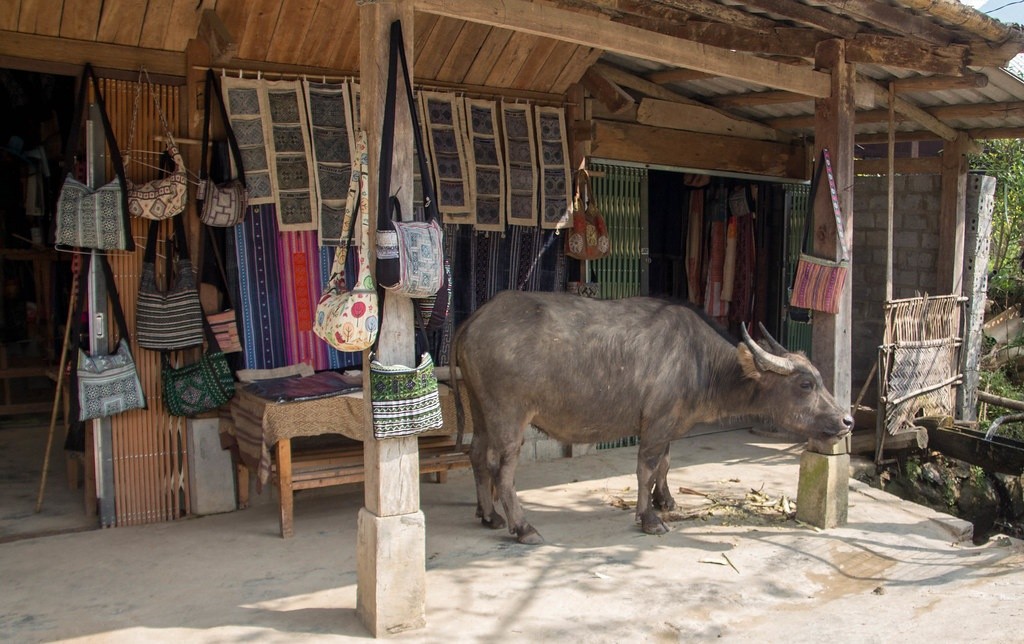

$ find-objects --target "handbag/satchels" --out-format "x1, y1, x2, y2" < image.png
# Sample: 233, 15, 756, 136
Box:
375, 219, 445, 300
197, 175, 249, 227
160, 350, 236, 416
203, 308, 244, 355
313, 273, 378, 353
121, 146, 187, 220
683, 172, 751, 220
136, 279, 204, 351
415, 259, 452, 332
564, 168, 611, 260
70, 336, 149, 422
790, 254, 849, 314
789, 273, 813, 324
54, 171, 136, 253
369, 352, 444, 442
566, 281, 601, 299
26, 174, 45, 216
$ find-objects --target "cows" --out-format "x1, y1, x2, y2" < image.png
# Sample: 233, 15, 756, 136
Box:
449, 289, 855, 545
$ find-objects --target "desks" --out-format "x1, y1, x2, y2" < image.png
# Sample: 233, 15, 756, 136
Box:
230, 379, 497, 540
